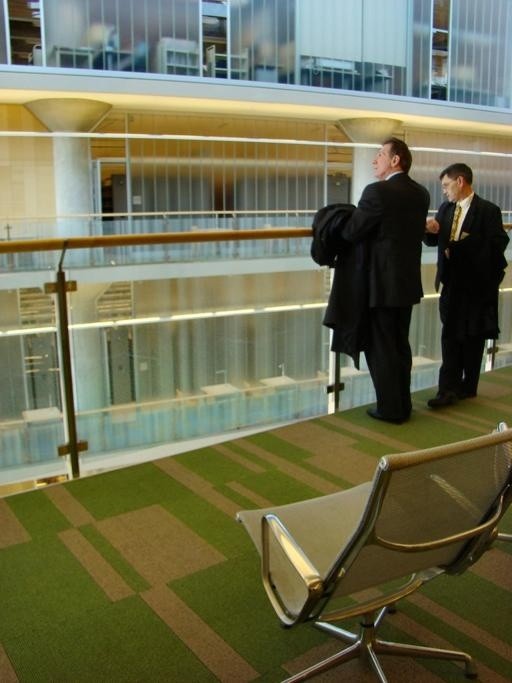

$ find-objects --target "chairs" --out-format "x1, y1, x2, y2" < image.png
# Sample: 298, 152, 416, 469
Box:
236, 429, 511, 682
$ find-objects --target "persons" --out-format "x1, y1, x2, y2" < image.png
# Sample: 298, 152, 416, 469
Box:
422, 161, 505, 408
329, 136, 431, 423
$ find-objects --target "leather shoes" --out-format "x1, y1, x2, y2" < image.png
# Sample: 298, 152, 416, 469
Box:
428, 390, 477, 407
367, 407, 402, 424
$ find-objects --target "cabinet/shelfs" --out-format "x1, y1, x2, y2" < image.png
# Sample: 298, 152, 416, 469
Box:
32, 22, 512, 113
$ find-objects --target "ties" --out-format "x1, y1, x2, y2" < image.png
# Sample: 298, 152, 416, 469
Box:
449, 205, 462, 241
